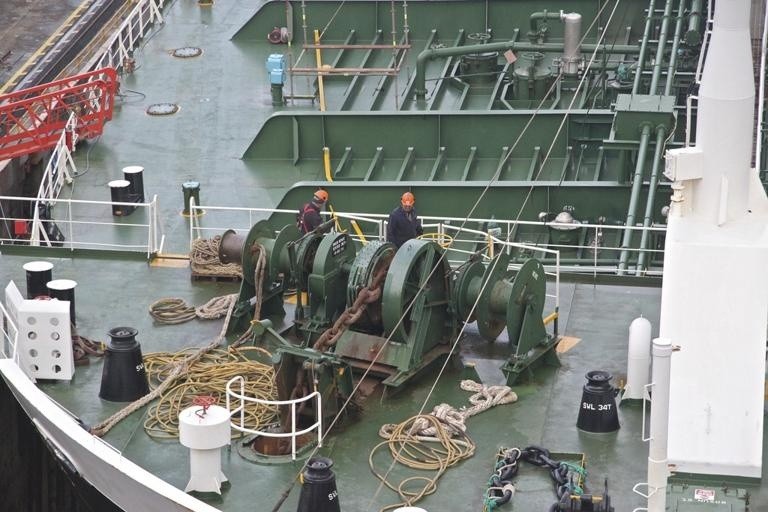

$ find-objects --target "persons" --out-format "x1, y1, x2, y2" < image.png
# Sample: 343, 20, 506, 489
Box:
387, 192, 424, 248
296, 189, 329, 236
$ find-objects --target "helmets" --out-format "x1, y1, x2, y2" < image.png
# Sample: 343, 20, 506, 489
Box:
400, 192, 415, 206
314, 190, 329, 202
